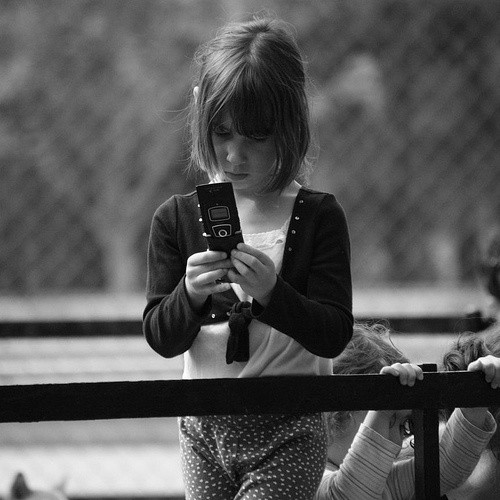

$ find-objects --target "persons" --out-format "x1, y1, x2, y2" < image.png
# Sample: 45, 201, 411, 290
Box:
143, 17, 353, 499
439, 320, 500, 500
310, 320, 500, 500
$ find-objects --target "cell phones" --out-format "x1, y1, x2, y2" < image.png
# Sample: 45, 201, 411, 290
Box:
195, 183, 245, 258
